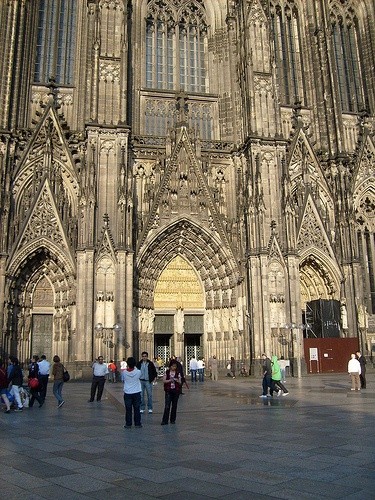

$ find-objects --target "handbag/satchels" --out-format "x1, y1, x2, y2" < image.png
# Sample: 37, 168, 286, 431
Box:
29, 377, 40, 388
63, 371, 70, 382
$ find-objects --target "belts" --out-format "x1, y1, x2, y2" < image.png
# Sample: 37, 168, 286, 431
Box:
139, 379, 146, 382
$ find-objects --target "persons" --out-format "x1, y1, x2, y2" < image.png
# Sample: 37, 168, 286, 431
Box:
28, 355, 50, 408
52, 355, 64, 408
190, 355, 205, 382
226, 357, 236, 378
210, 355, 218, 381
108, 360, 120, 383
168, 356, 188, 389
161, 359, 182, 425
153, 357, 162, 386
0, 356, 23, 413
88, 356, 107, 402
136, 352, 156, 414
348, 351, 366, 390
259, 353, 289, 398
122, 357, 143, 428
120, 358, 128, 383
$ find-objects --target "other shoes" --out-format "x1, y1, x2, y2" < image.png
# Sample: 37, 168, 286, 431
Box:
276, 390, 281, 397
351, 388, 356, 390
135, 423, 144, 428
147, 409, 153, 413
170, 420, 175, 424
38, 400, 44, 408
267, 393, 272, 396
56, 400, 64, 408
29, 405, 33, 408
161, 422, 168, 425
259, 394, 267, 398
4, 410, 11, 414
14, 406, 23, 412
357, 387, 360, 390
282, 392, 289, 396
139, 409, 145, 413
124, 424, 131, 429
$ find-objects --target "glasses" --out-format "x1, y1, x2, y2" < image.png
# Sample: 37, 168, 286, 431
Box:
142, 356, 146, 357
